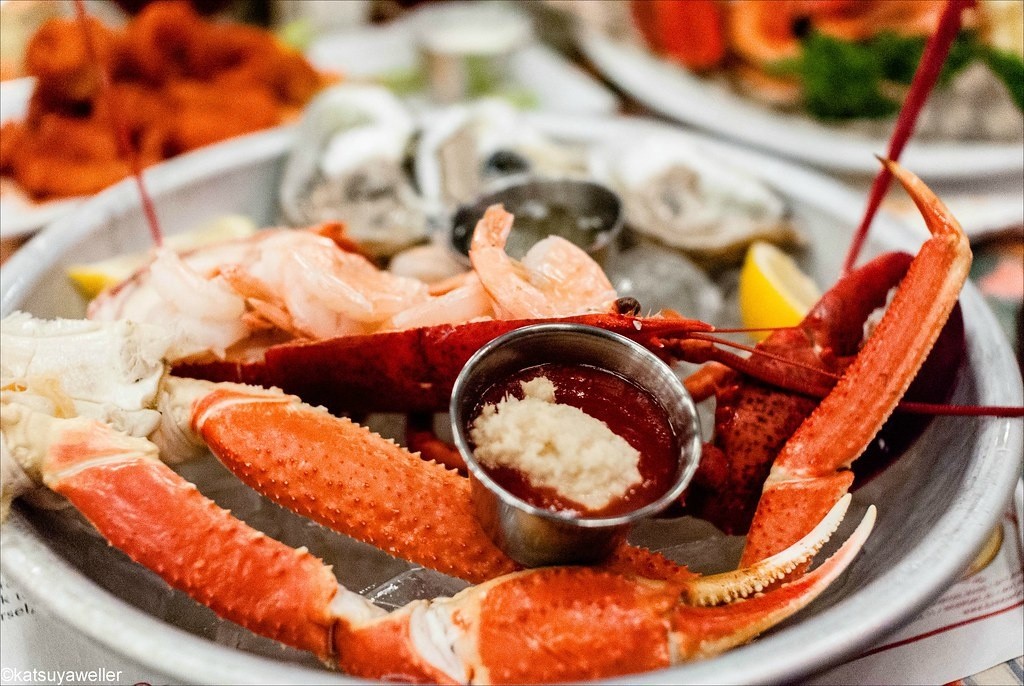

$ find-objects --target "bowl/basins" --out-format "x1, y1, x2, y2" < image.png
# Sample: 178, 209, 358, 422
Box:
448, 322, 699, 565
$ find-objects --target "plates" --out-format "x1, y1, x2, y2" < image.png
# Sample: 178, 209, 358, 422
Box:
565, 0, 1024, 179
1, 8, 616, 253
1, 107, 1024, 683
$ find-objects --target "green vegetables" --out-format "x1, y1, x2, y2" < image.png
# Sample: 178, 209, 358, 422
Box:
768, 29, 1024, 122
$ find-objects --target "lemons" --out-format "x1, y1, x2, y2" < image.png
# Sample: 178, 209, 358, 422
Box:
67, 215, 254, 300
741, 238, 822, 341
